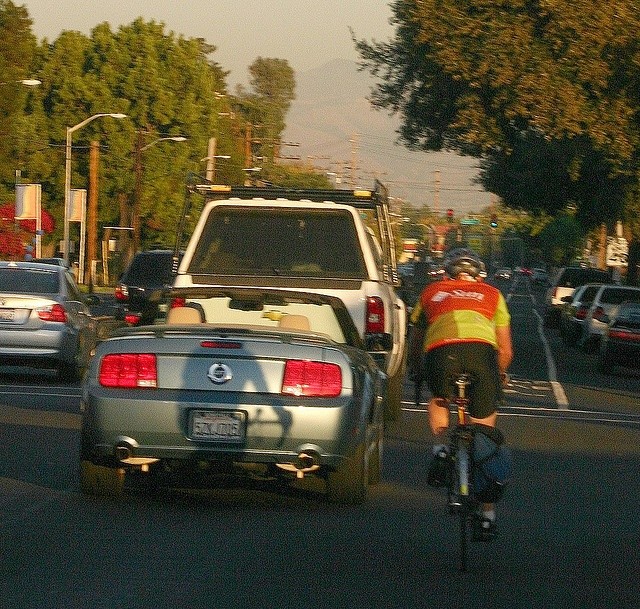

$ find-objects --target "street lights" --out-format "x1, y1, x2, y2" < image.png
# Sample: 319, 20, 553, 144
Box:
2, 78, 42, 87
134, 131, 187, 250
201, 157, 232, 204
64, 112, 128, 265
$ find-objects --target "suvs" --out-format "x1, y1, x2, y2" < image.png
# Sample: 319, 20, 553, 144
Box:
541, 267, 612, 326
161, 171, 408, 422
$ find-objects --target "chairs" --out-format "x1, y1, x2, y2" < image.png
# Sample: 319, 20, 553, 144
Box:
280, 315, 310, 331
166, 306, 201, 326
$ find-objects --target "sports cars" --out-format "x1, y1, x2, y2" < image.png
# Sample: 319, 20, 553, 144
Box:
79, 286, 388, 505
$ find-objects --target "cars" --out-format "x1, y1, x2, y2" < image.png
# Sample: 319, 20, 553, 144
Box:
0, 260, 98, 383
495, 269, 513, 280
560, 283, 601, 345
533, 269, 549, 282
113, 250, 185, 326
598, 302, 637, 376
582, 284, 640, 350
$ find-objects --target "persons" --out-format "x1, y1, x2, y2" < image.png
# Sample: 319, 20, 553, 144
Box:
409, 249, 514, 541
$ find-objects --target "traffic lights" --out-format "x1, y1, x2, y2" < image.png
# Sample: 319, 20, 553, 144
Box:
445, 208, 453, 225
490, 214, 498, 228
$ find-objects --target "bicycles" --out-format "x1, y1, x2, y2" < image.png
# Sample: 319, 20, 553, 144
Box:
410, 370, 505, 573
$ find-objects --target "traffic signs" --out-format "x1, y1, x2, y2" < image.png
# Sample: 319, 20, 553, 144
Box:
461, 218, 480, 225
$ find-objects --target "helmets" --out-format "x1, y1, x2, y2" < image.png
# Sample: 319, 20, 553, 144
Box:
444, 248, 482, 276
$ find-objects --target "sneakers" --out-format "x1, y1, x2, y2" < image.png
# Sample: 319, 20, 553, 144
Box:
476, 522, 499, 538
428, 451, 451, 489
